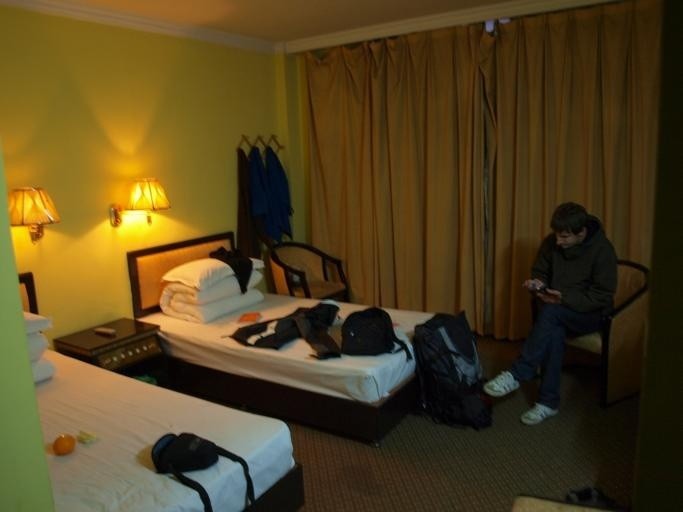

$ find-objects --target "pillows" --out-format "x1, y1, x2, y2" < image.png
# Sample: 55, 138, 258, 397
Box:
22, 309, 52, 334
159, 254, 265, 291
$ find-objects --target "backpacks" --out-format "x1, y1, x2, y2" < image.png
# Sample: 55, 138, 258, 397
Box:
412, 310, 493, 427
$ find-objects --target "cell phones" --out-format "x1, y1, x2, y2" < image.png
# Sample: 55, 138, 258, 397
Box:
529, 284, 547, 296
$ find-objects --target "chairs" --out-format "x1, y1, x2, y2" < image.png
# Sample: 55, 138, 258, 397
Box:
270, 241, 350, 304
563, 258, 649, 403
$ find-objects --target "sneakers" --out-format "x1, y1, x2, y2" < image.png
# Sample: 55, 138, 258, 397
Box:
520, 402, 560, 426
483, 368, 521, 397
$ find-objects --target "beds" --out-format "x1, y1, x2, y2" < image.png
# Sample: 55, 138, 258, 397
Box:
126, 230, 438, 450
16, 269, 304, 512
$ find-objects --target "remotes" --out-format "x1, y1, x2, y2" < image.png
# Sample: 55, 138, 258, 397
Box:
94, 327, 115, 335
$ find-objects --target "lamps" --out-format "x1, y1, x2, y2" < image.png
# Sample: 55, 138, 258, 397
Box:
7, 185, 60, 245
108, 176, 170, 229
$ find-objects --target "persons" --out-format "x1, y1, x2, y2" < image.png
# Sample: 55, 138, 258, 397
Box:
482, 201, 618, 426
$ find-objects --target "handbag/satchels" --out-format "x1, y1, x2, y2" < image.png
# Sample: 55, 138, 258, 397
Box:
341, 307, 394, 356
151, 433, 217, 473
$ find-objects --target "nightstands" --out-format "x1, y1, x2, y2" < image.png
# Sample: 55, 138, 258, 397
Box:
52, 317, 168, 388
